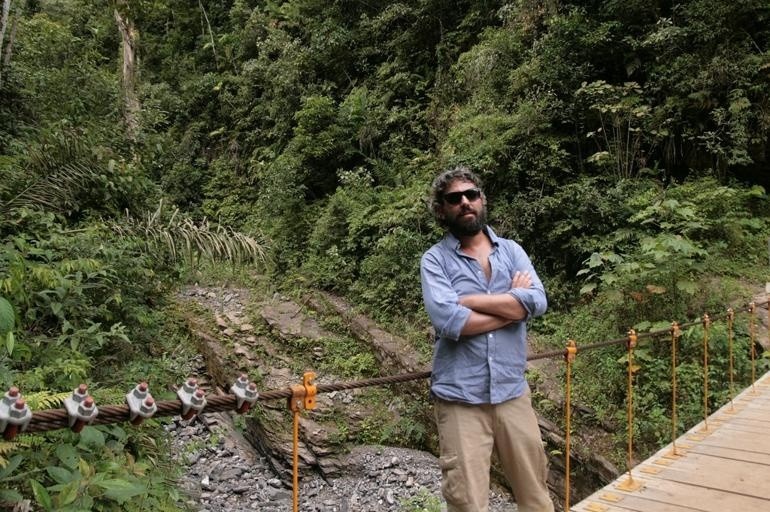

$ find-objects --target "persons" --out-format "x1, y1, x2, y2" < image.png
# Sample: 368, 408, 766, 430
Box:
420, 164, 555, 512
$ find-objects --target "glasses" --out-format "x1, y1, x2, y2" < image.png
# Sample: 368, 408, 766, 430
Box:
442, 188, 481, 205
